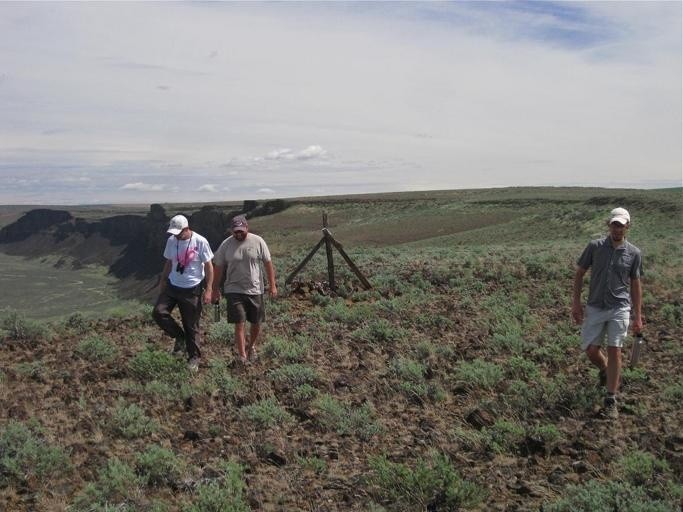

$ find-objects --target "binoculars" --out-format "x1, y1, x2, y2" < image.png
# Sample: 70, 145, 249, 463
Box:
176, 263, 184, 274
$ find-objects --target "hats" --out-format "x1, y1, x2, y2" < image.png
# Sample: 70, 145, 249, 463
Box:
608, 206, 631, 225
231, 216, 248, 232
166, 215, 189, 236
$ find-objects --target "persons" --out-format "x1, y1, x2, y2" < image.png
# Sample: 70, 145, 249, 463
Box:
571, 206, 644, 421
210, 214, 277, 358
150, 214, 214, 373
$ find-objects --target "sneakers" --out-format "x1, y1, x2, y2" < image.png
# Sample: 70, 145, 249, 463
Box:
249, 344, 258, 363
599, 368, 609, 395
173, 332, 200, 373
604, 397, 619, 419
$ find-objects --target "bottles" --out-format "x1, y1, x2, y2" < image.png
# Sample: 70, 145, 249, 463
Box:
213, 302, 221, 323
631, 333, 643, 366
